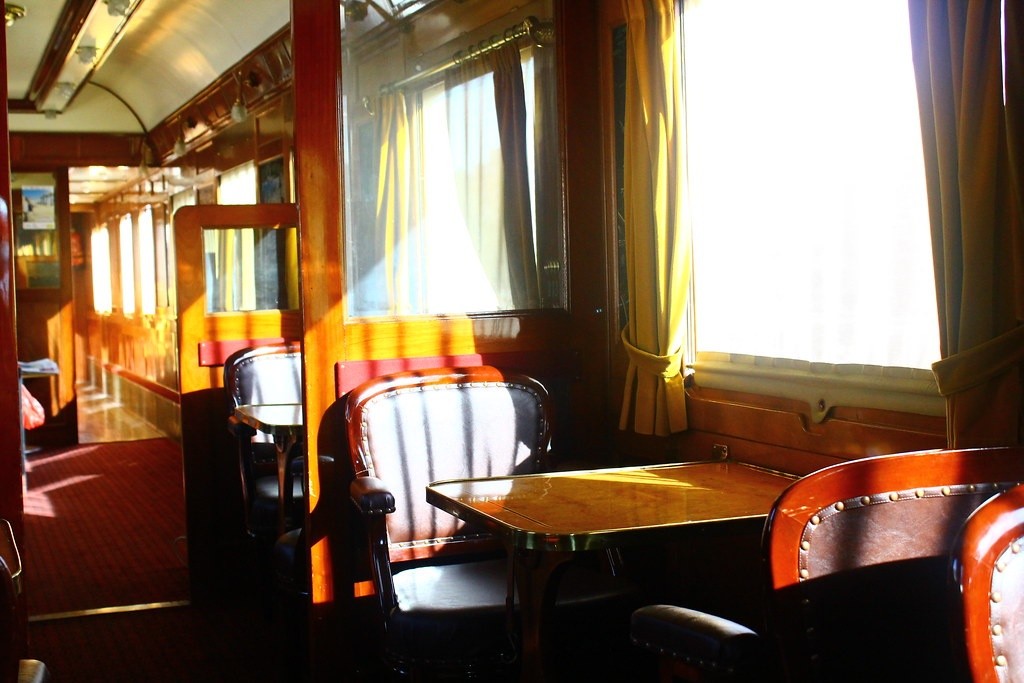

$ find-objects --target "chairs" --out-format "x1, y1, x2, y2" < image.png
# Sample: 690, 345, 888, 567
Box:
334, 371, 554, 683
226, 342, 303, 535
622, 441, 1024, 683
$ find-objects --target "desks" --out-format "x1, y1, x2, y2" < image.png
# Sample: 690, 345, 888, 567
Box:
235, 402, 302, 538
427, 458, 808, 683
